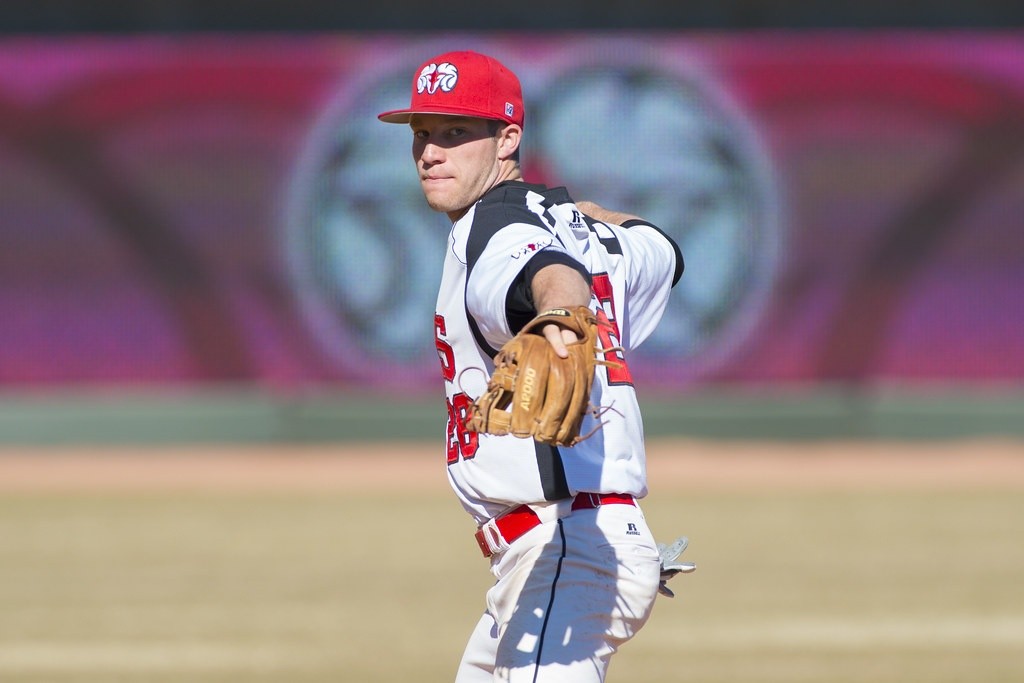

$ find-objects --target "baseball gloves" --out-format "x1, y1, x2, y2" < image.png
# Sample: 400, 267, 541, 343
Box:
457, 304, 626, 451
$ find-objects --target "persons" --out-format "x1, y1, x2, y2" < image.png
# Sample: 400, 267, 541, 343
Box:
378, 49, 684, 683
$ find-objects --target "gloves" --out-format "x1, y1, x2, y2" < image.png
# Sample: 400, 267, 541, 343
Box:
654, 537, 698, 599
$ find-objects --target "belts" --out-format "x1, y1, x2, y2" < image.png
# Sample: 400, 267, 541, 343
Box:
473, 492, 637, 558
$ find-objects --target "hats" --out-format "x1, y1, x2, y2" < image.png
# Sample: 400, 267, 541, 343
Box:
375, 49, 526, 132
